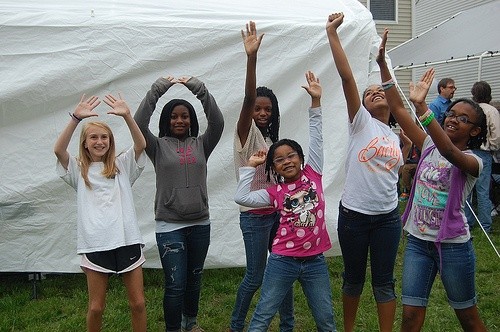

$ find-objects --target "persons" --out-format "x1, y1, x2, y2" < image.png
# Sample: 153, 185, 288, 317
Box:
376, 27, 488, 332
428, 78, 457, 130
229, 21, 294, 332
234, 70, 337, 332
397, 143, 421, 203
133, 76, 224, 332
489, 151, 500, 216
326, 11, 413, 332
54, 92, 147, 332
464, 81, 500, 234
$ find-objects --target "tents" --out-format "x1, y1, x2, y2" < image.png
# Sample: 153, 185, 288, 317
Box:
0, 0, 397, 273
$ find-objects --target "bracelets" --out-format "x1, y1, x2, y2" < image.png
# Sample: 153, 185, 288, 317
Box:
419, 109, 434, 127
68, 112, 82, 123
382, 79, 395, 90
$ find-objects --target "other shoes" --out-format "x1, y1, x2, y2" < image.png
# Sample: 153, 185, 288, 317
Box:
489, 204, 500, 216
398, 192, 410, 202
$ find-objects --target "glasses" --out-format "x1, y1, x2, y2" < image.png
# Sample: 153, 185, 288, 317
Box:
272, 150, 299, 164
444, 86, 456, 90
444, 110, 481, 127
